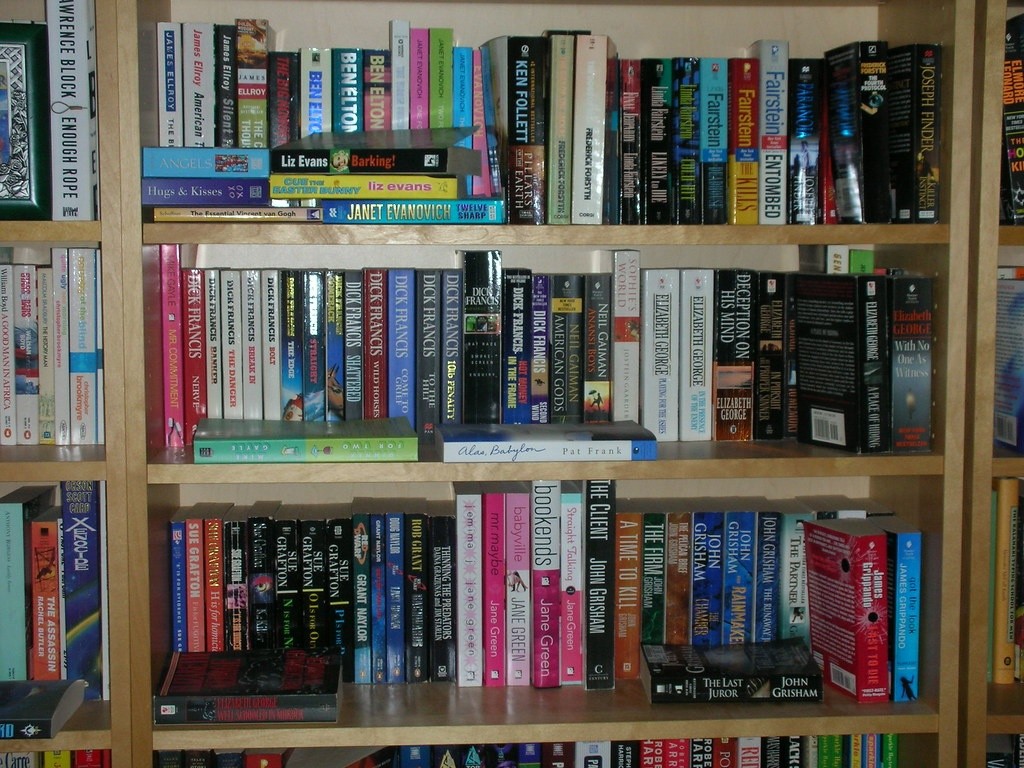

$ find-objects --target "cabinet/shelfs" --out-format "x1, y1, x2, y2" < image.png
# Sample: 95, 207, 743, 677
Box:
115, 0, 975, 768
0, 0, 134, 768
958, 0, 1024, 768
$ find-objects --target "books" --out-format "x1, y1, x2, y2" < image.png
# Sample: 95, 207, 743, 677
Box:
0, 16, 1024, 768
47, 2, 102, 222
